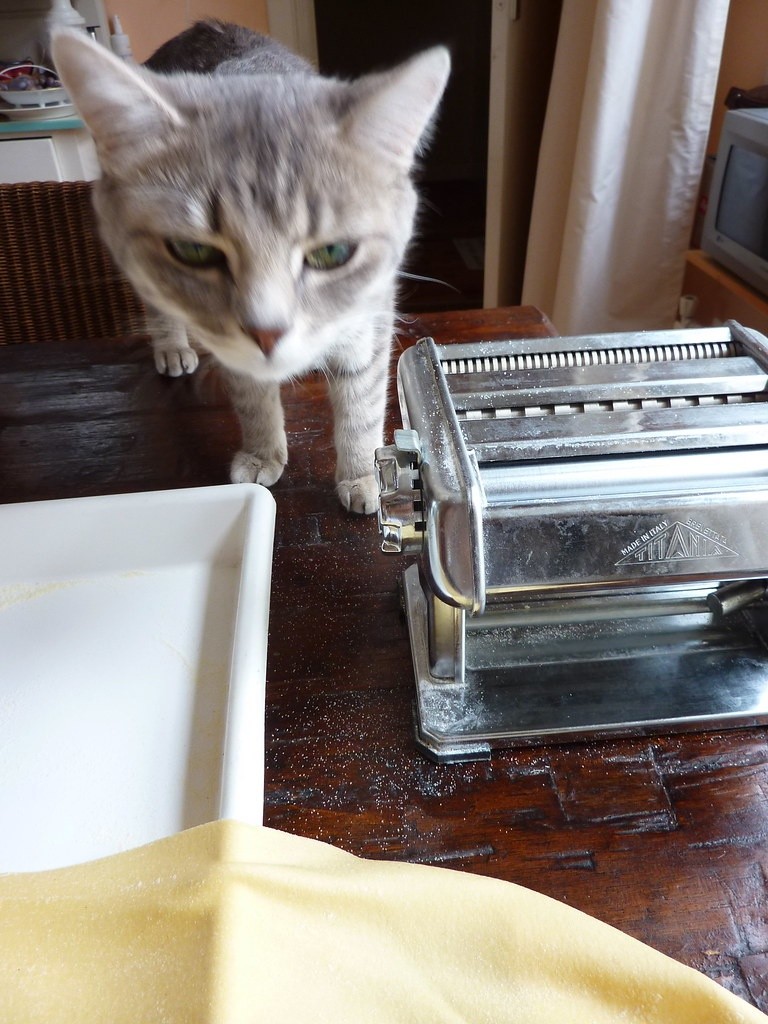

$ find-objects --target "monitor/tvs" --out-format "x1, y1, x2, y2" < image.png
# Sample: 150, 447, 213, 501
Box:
701, 106, 768, 295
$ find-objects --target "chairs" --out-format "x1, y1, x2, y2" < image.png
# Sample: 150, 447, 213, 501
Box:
0, 181, 148, 344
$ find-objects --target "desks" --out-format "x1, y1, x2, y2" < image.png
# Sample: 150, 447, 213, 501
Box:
0, 303, 768, 1014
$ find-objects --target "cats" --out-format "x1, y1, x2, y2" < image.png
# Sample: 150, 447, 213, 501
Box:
48, 16, 452, 515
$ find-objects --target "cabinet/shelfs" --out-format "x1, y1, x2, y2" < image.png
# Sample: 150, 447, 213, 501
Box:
673, 249, 768, 339
0, 114, 103, 184
